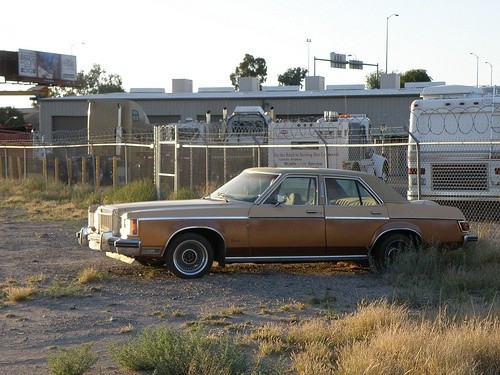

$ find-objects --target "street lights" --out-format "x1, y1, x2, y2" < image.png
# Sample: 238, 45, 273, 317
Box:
384, 13, 401, 74
485, 61, 493, 86
470, 52, 479, 87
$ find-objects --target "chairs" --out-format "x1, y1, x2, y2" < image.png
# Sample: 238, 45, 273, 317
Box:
285, 193, 300, 205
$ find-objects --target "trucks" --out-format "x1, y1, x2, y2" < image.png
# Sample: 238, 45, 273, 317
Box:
404, 85, 500, 200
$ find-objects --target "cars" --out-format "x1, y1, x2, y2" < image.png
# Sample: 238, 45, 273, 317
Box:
76, 166, 479, 282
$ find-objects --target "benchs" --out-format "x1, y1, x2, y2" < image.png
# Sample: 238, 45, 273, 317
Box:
335, 197, 377, 206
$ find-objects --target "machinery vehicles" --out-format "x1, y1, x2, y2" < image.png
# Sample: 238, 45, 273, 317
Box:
164, 106, 390, 182
